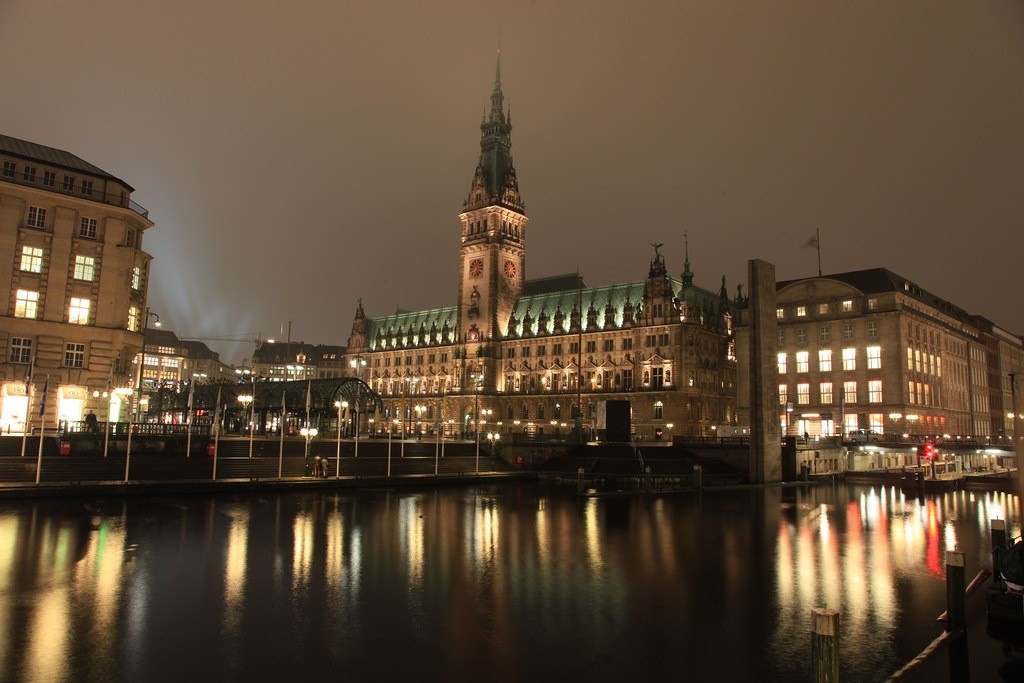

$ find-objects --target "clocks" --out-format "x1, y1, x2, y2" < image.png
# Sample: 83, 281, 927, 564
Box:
502, 256, 517, 278
468, 255, 486, 280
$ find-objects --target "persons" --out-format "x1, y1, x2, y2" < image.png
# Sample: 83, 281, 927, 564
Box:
417, 426, 422, 440
314, 453, 322, 478
321, 456, 329, 478
85, 409, 97, 435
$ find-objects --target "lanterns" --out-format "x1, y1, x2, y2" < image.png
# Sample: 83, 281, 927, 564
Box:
207, 443, 215, 456
60, 441, 71, 457
517, 455, 522, 464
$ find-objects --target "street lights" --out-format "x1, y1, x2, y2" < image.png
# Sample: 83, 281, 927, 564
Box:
488, 433, 501, 472
448, 419, 454, 438
300, 428, 318, 477
335, 401, 348, 479
134, 307, 161, 436
187, 370, 208, 458
415, 406, 427, 440
666, 423, 673, 441
237, 395, 253, 437
481, 409, 492, 438
561, 422, 567, 440
551, 420, 557, 432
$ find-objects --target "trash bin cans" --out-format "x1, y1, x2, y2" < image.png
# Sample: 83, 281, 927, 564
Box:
800, 460, 807, 481
116, 422, 124, 435
131, 426, 138, 435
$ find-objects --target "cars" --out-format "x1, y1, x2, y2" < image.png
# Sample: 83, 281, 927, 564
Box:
847, 428, 885, 443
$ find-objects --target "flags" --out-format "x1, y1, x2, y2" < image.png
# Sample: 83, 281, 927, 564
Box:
25, 355, 35, 394
282, 395, 286, 416
306, 380, 312, 413
39, 375, 50, 417
213, 387, 221, 432
188, 374, 195, 408
106, 367, 113, 393
356, 387, 360, 414
